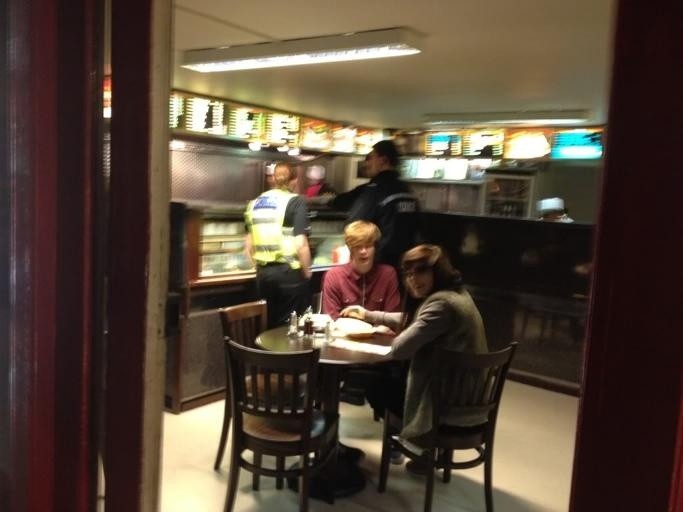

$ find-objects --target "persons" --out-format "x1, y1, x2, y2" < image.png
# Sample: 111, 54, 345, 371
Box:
320, 218, 402, 322
265, 140, 426, 267
534, 197, 577, 223
242, 162, 315, 326
338, 243, 490, 476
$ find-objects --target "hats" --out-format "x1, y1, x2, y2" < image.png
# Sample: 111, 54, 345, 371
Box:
306, 164, 325, 180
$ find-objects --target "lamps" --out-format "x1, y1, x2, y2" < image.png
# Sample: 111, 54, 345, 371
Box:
181, 24, 424, 74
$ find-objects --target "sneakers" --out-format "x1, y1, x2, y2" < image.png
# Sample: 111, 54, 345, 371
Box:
390, 445, 446, 474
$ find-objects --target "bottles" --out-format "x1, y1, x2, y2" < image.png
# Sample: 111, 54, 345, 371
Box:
304, 317, 314, 337
502, 203, 518, 217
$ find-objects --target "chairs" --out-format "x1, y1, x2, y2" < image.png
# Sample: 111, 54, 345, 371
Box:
209, 298, 346, 512
377, 339, 521, 511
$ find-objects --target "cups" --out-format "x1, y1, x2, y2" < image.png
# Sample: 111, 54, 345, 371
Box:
287, 317, 300, 337
